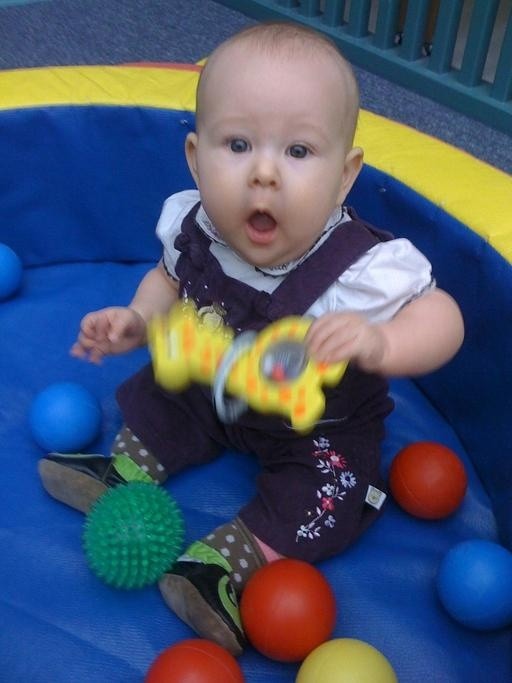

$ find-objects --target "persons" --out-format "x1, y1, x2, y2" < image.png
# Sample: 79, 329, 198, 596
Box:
37, 22, 466, 658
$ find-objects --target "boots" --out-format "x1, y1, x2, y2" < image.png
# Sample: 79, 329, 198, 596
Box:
157, 512, 268, 659
38, 422, 171, 517
